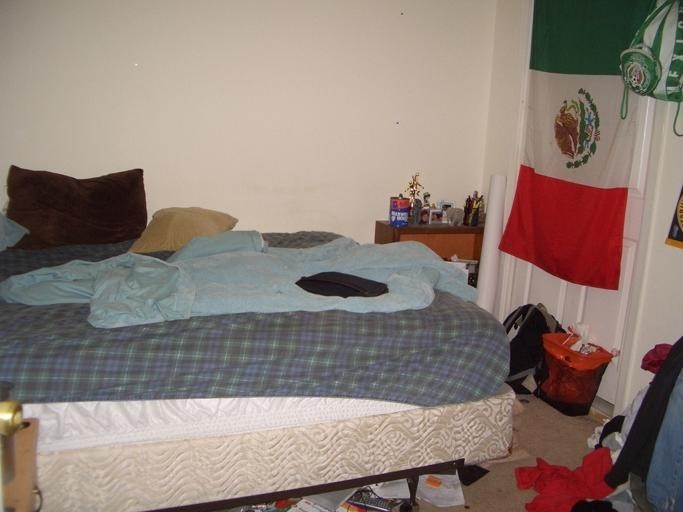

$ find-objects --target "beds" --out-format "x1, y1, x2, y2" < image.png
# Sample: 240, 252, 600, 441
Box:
1, 230, 516, 511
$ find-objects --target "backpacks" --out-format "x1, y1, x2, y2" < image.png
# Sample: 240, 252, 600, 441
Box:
614, 0, 683, 135
502, 304, 564, 395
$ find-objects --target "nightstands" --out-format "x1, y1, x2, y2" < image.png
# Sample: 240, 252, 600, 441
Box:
374, 220, 485, 288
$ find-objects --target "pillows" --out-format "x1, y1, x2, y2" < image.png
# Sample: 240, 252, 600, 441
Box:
129, 206, 239, 255
6, 163, 148, 249
